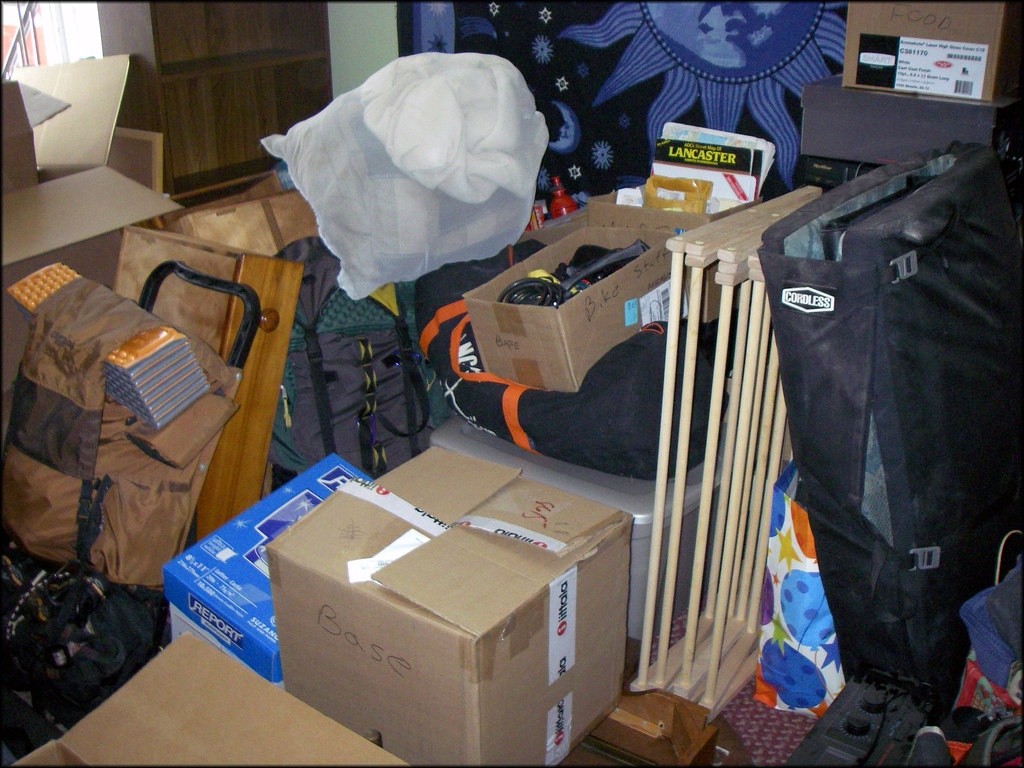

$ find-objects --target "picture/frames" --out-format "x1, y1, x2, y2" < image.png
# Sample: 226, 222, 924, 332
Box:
106, 128, 163, 196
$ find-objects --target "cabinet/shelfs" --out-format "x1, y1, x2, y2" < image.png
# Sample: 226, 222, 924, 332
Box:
101, 0, 334, 207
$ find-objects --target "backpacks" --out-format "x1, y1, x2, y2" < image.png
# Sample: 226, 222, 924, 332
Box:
1, 277, 243, 730
267, 237, 451, 479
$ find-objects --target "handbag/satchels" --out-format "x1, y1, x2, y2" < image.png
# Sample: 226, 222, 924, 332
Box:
857, 529, 1023, 768
752, 457, 847, 717
414, 238, 743, 478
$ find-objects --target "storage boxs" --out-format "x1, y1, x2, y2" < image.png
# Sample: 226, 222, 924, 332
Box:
841, 0, 1010, 103
7, 629, 410, 768
587, 183, 762, 324
0, 52, 184, 418
263, 445, 640, 767
162, 454, 376, 694
462, 224, 690, 396
801, 72, 1009, 166
430, 393, 731, 645
